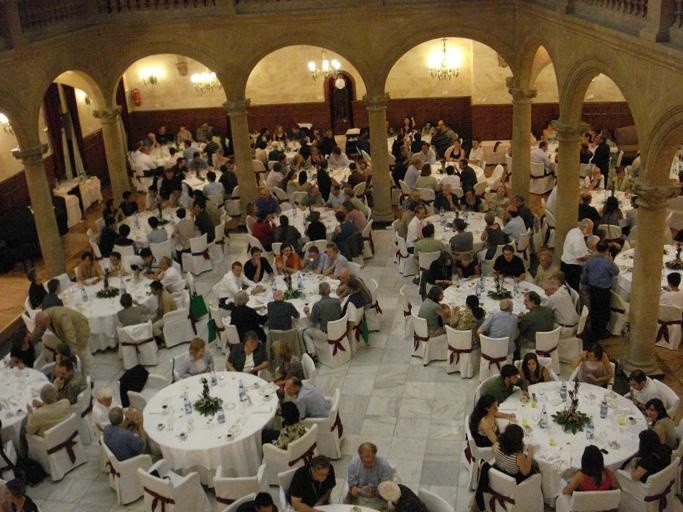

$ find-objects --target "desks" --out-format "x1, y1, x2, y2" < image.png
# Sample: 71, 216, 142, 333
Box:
0, 196, 67, 273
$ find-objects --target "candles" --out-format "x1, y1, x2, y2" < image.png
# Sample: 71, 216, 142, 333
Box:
77, 157, 82, 180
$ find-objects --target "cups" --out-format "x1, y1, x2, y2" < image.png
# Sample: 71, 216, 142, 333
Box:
627, 417, 636, 426
16, 408, 22, 413
161, 405, 169, 412
264, 395, 271, 400
252, 383, 259, 389
217, 373, 223, 387
178, 432, 188, 440
521, 396, 528, 405
621, 255, 626, 259
476, 285, 483, 298
226, 434, 234, 441
309, 258, 315, 263
156, 423, 165, 431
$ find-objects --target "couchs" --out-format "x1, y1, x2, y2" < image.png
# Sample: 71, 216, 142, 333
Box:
614, 125, 641, 163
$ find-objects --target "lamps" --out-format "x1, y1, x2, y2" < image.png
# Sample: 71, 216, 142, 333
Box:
0, 113, 13, 136
306, 47, 342, 81
187, 68, 221, 94
137, 66, 165, 87
427, 38, 462, 81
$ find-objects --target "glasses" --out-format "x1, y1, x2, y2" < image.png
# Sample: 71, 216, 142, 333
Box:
314, 469, 330, 476
283, 249, 292, 253
503, 252, 513, 256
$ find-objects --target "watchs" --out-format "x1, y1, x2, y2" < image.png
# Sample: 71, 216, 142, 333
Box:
250, 366, 255, 372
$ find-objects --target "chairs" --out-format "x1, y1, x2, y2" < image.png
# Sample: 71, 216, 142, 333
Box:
579, 163, 594, 185
479, 160, 485, 170
137, 458, 210, 512
417, 488, 454, 512
25, 413, 89, 482
219, 492, 255, 512
485, 163, 502, 198
622, 378, 679, 425
250, 159, 262, 183
409, 306, 448, 367
116, 370, 168, 409
418, 250, 440, 294
362, 204, 370, 218
23, 292, 42, 319
503, 150, 511, 187
361, 218, 374, 259
399, 282, 421, 338
345, 298, 367, 356
147, 239, 176, 270
207, 220, 225, 263
353, 182, 368, 205
206, 193, 225, 213
211, 465, 266, 509
304, 388, 344, 460
70, 375, 101, 447
297, 123, 311, 130
162, 289, 195, 349
515, 226, 532, 270
670, 419, 682, 496
344, 231, 364, 268
212, 135, 223, 154
613, 457, 679, 512
85, 413, 106, 473
261, 180, 279, 202
246, 235, 271, 258
220, 315, 241, 361
462, 414, 493, 491
261, 423, 317, 486
85, 227, 98, 243
94, 216, 104, 233
558, 305, 588, 364
513, 357, 558, 383
73, 261, 106, 282
343, 461, 397, 505
467, 460, 543, 512
171, 257, 181, 274
126, 152, 135, 185
355, 146, 362, 160
123, 255, 145, 273
271, 243, 295, 268
211, 281, 221, 300
223, 352, 262, 376
389, 170, 400, 206
115, 317, 159, 369
444, 323, 478, 379
607, 150, 623, 174
520, 325, 561, 377
597, 222, 622, 255
413, 189, 435, 213
134, 163, 146, 190
269, 185, 289, 202
528, 160, 554, 193
388, 151, 396, 171
569, 357, 615, 390
208, 304, 231, 348
20, 313, 62, 354
4, 350, 46, 371
43, 272, 76, 296
395, 230, 416, 277
274, 354, 316, 391
473, 374, 521, 410
98, 258, 129, 274
542, 208, 556, 247
277, 463, 300, 512
219, 212, 230, 246
539, 196, 545, 228
391, 218, 400, 264
471, 181, 485, 198
365, 278, 383, 331
169, 351, 190, 382
554, 490, 620, 512
301, 240, 327, 255
477, 239, 513, 274
550, 163, 558, 187
267, 329, 306, 379
248, 132, 260, 144
183, 271, 196, 298
564, 282, 579, 315
348, 260, 362, 279
664, 211, 674, 244
181, 232, 212, 276
449, 248, 478, 278
87, 238, 107, 262
291, 191, 307, 206
396, 179, 412, 204
654, 304, 681, 350
450, 187, 463, 202
312, 313, 351, 369
479, 334, 512, 380
266, 160, 280, 169
129, 158, 136, 187
225, 185, 241, 214
606, 288, 629, 336
113, 245, 135, 258
42, 355, 81, 379
459, 136, 462, 146
99, 432, 153, 505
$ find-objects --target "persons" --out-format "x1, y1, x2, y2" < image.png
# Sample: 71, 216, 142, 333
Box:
598, 196, 623, 225
268, 163, 280, 187
171, 209, 195, 260
290, 125, 305, 142
42, 279, 63, 307
563, 445, 621, 494
403, 158, 423, 188
518, 291, 553, 348
92, 389, 136, 428
342, 202, 367, 229
285, 377, 330, 420
583, 167, 604, 190
417, 287, 450, 337
312, 169, 337, 203
399, 201, 414, 239
299, 187, 321, 210
255, 141, 267, 171
119, 191, 138, 219
580, 193, 598, 220
394, 146, 407, 173
405, 206, 430, 254
342, 187, 366, 215
219, 261, 264, 306
192, 191, 219, 224
29, 307, 88, 354
493, 246, 525, 283
485, 364, 530, 404
268, 216, 301, 249
251, 212, 274, 251
150, 282, 178, 350
4, 479, 37, 511
624, 196, 639, 234
169, 186, 193, 208
261, 401, 305, 450
411, 133, 425, 151
277, 243, 299, 274
279, 154, 288, 175
360, 160, 371, 187
226, 332, 269, 375
447, 140, 464, 166
170, 158, 189, 178
386, 121, 393, 137
465, 139, 482, 167
203, 135, 220, 166
332, 270, 371, 313
145, 185, 162, 210
361, 127, 370, 155
536, 252, 559, 288
542, 283, 579, 338
28, 270, 46, 307
428, 252, 453, 290
230, 292, 267, 341
493, 424, 539, 484
421, 122, 435, 137
438, 120, 459, 142
629, 370, 668, 414
254, 187, 280, 217
378, 483, 428, 511
167, 147, 179, 168
134, 248, 160, 278
117, 293, 151, 327
10, 331, 38, 368
247, 203, 256, 235
77, 252, 104, 285
461, 189, 484, 212
57, 360, 87, 402
506, 195, 532, 229
560, 218, 593, 288
154, 256, 187, 293
99, 216, 118, 256
322, 130, 340, 154
530, 140, 551, 175
581, 241, 619, 339
103, 198, 121, 223
658, 272, 683, 308
393, 134, 412, 151
519, 353, 549, 384
347, 443, 393, 511
189, 153, 207, 171
103, 409, 146, 458
456, 251, 480, 278
646, 399, 677, 448
311, 144, 325, 166
235, 492, 277, 512
500, 205, 525, 251
201, 172, 224, 207
324, 245, 347, 278
306, 246, 330, 274
104, 252, 131, 277
27, 384, 70, 436
592, 135, 609, 189
433, 129, 451, 158
256, 128, 271, 149
580, 143, 593, 163
449, 295, 485, 346
342, 280, 363, 338
226, 159, 235, 174
335, 211, 356, 245
269, 289, 299, 329
459, 160, 478, 189
471, 395, 514, 444
148, 217, 167, 247
584, 235, 620, 259
273, 124, 287, 150
157, 126, 172, 142
477, 299, 520, 354
401, 117, 415, 134
292, 171, 315, 202
160, 170, 181, 193
435, 183, 457, 212
449, 218, 473, 252
129, 143, 141, 173
47, 343, 81, 376
144, 132, 159, 150
279, 171, 295, 193
195, 122, 212, 141
184, 140, 197, 161
413, 142, 435, 164
268, 341, 304, 387
551, 270, 577, 310
292, 145, 311, 170
136, 145, 165, 188
485, 185, 509, 212
285, 455, 336, 511
631, 429, 672, 482
245, 247, 273, 283
304, 211, 326, 238
219, 165, 236, 198
413, 163, 439, 210
181, 338, 214, 378
327, 147, 348, 169
303, 284, 341, 363
115, 223, 140, 255
481, 213, 501, 259
177, 126, 191, 144
340, 162, 364, 199
583, 126, 600, 146
190, 199, 214, 241
573, 343, 612, 387
414, 223, 446, 284
547, 179, 557, 213
441, 167, 461, 186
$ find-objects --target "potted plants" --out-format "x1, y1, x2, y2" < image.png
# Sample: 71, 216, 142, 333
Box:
549, 377, 593, 435
96, 268, 119, 299
445, 211, 469, 232
192, 377, 222, 417
154, 199, 169, 225
487, 271, 513, 300
281, 271, 302, 300
664, 241, 682, 270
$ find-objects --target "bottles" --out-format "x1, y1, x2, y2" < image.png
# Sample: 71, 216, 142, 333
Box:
513, 278, 518, 296
80, 285, 88, 303
296, 272, 303, 290
168, 212, 175, 227
586, 416, 594, 440
237, 379, 247, 401
480, 273, 485, 292
475, 281, 481, 298
539, 405, 547, 427
291, 204, 296, 219
560, 378, 567, 398
600, 394, 608, 417
462, 209, 468, 222
604, 188, 609, 201
183, 393, 191, 415
119, 277, 126, 295
217, 400, 225, 424
209, 363, 216, 386
439, 210, 445, 226
271, 279, 276, 294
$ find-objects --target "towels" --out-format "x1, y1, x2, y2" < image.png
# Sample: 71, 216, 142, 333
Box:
229, 423, 239, 439
150, 403, 171, 416
252, 402, 273, 413
